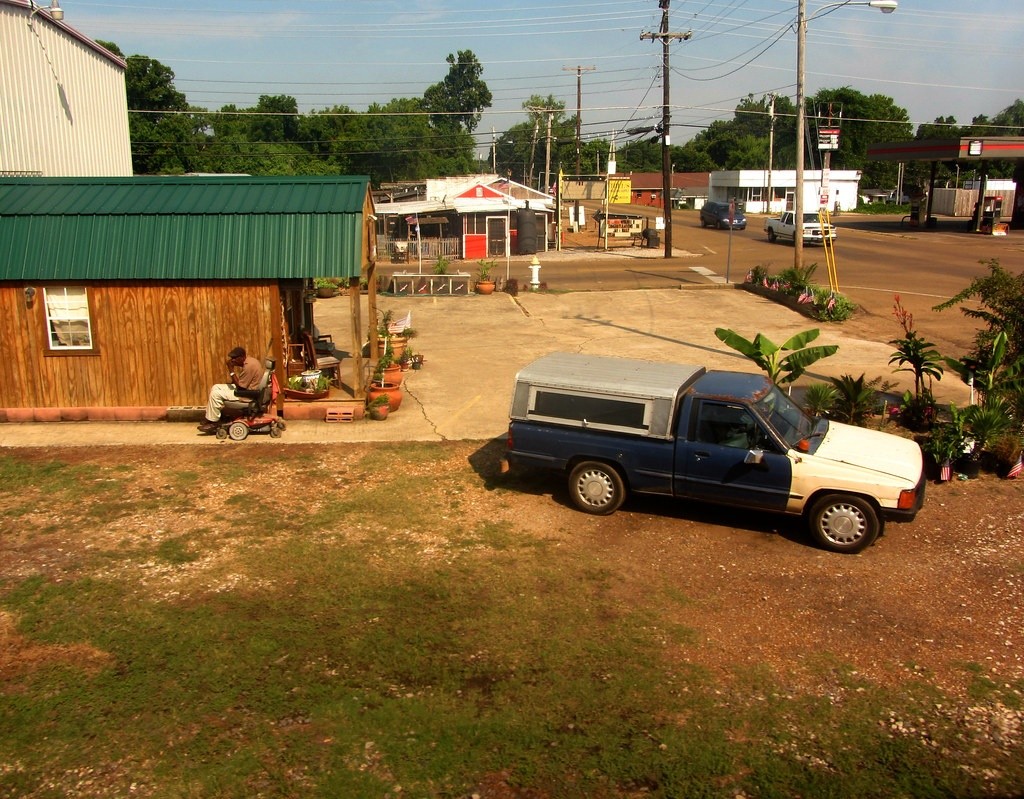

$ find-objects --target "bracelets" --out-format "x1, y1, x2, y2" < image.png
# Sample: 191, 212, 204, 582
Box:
230, 373, 235, 376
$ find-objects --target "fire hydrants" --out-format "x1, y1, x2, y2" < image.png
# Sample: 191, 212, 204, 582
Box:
527, 256, 541, 291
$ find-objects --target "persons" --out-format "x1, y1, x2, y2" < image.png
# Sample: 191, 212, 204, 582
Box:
197, 347, 264, 433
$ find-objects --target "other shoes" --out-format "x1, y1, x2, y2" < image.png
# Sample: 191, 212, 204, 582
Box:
198, 422, 217, 432
200, 418, 215, 425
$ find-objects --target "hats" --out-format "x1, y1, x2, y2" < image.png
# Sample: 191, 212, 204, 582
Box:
228, 347, 246, 358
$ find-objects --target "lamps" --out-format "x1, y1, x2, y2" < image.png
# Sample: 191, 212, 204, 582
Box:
24, 286, 36, 303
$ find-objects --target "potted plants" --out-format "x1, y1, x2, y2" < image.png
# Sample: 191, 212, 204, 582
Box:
474, 260, 498, 295
317, 282, 338, 297
432, 257, 450, 280
285, 376, 331, 400
368, 309, 423, 421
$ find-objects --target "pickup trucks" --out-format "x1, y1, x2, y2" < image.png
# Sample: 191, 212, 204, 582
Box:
763, 209, 838, 247
500, 354, 927, 555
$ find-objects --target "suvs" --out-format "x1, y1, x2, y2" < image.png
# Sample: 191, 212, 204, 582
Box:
700, 200, 748, 230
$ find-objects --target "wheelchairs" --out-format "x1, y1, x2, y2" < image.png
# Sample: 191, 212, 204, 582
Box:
207, 357, 287, 440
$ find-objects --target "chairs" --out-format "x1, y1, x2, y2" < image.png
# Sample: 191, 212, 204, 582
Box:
300, 330, 343, 390
224, 359, 275, 409
307, 323, 335, 357
391, 241, 410, 264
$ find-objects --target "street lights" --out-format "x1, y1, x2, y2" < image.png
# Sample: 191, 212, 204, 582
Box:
493, 140, 513, 175
792, 0, 897, 270
671, 164, 675, 188
954, 164, 960, 188
528, 135, 558, 188
